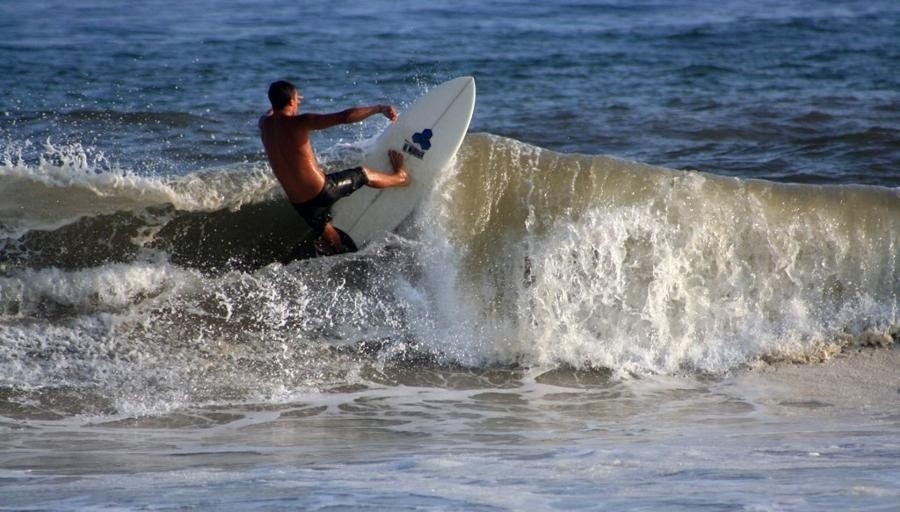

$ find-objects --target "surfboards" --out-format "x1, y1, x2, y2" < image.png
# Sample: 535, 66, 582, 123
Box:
329, 76, 476, 249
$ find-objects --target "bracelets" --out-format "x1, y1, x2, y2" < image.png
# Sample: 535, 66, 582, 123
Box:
376, 103, 383, 114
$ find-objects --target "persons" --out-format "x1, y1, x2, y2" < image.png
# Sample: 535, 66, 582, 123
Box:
256, 77, 411, 255
